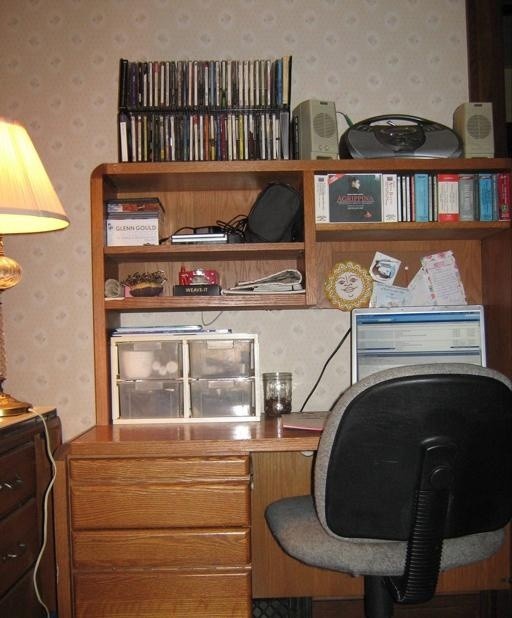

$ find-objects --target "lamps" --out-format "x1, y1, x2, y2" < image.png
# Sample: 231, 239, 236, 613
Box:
0, 120, 71, 421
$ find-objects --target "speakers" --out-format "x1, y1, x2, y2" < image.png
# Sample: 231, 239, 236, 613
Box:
453, 101, 495, 158
292, 100, 340, 160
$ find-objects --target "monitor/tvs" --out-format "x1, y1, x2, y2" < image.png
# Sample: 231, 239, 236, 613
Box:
350, 305, 487, 387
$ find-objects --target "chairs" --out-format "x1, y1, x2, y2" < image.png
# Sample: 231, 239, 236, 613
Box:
262, 362, 512, 618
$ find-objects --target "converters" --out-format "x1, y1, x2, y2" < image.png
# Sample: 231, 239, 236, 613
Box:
194, 223, 222, 234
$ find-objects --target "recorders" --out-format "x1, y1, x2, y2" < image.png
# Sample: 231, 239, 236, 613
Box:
341, 113, 463, 158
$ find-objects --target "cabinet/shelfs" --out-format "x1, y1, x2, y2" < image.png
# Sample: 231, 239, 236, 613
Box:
52, 157, 512, 618
0, 401, 62, 617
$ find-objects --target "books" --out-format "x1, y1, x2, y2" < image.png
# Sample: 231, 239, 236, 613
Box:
224, 290, 306, 295
474, 174, 479, 220
314, 174, 330, 225
498, 173, 512, 222
433, 177, 438, 222
397, 177, 414, 222
253, 285, 302, 291
381, 173, 397, 223
415, 174, 428, 223
328, 174, 380, 224
493, 174, 499, 222
116, 55, 292, 110
118, 112, 300, 162
437, 174, 460, 222
171, 233, 228, 238
479, 174, 492, 222
458, 173, 475, 222
105, 197, 165, 213
428, 176, 433, 223
171, 238, 229, 243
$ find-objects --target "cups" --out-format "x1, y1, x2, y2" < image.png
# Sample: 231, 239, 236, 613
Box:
263, 373, 291, 417
